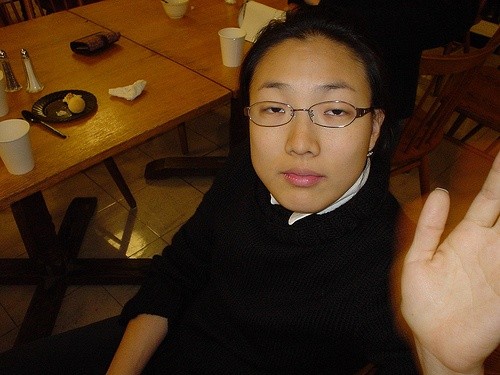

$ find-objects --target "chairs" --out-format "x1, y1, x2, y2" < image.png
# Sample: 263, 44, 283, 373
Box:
389, 27, 500, 195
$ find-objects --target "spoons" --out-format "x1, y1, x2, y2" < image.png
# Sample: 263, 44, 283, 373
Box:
22, 109, 67, 138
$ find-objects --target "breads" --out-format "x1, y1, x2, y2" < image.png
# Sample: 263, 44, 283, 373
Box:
108, 80, 147, 101
62, 93, 85, 113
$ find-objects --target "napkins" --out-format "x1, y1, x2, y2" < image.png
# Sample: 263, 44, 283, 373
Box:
109, 80, 148, 101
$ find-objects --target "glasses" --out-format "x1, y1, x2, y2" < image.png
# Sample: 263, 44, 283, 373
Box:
243, 101, 372, 128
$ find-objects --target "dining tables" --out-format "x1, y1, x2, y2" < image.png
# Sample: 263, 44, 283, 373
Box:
0, 11, 233, 349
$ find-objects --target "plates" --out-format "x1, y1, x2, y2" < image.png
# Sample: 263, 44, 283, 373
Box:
31, 89, 97, 123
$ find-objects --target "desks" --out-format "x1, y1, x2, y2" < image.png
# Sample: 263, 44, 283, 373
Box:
67, 0, 297, 179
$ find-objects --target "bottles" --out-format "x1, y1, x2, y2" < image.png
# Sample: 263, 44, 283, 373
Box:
20, 49, 44, 94
0, 50, 22, 93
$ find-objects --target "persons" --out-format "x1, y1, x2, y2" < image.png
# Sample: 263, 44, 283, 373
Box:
0, 10, 500, 375
285, 0, 479, 125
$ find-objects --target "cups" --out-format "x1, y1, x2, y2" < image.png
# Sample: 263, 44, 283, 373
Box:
218, 27, 248, 68
160, 0, 189, 19
0, 119, 35, 176
0, 69, 10, 117
